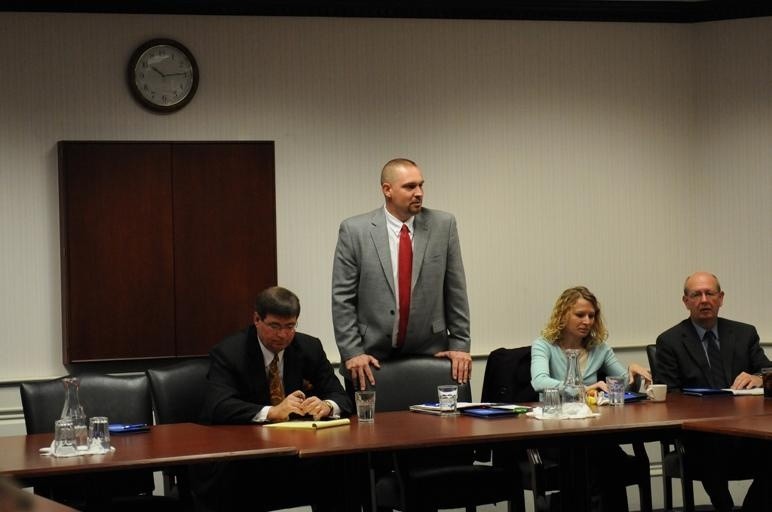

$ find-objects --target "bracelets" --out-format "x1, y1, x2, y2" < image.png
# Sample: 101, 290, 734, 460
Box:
323, 399, 333, 417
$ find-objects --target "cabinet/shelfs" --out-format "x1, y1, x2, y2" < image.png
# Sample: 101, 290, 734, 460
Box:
55, 136, 281, 369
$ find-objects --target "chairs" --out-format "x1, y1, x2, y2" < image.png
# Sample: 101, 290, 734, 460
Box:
147, 360, 325, 511
481, 346, 655, 511
647, 343, 706, 510
337, 353, 527, 512
17, 369, 189, 510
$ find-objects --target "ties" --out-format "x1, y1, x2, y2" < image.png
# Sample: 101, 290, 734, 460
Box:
269, 354, 290, 423
704, 330, 728, 389
397, 225, 412, 348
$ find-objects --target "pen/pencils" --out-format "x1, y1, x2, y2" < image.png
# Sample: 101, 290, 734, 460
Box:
123, 424, 147, 429
426, 403, 440, 406
683, 392, 703, 397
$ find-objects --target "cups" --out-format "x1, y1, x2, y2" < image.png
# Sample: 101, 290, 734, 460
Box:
606, 374, 625, 406
88, 415, 112, 454
53, 420, 78, 454
437, 385, 458, 416
646, 384, 667, 402
541, 387, 561, 416
762, 368, 772, 399
354, 391, 377, 424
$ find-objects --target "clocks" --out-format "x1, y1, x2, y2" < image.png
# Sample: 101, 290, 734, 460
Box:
124, 34, 203, 117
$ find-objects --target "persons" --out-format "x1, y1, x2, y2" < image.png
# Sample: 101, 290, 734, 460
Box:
330, 155, 473, 407
175, 287, 357, 510
524, 283, 653, 511
654, 269, 772, 512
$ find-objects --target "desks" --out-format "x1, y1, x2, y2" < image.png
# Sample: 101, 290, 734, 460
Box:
268, 393, 771, 459
2, 419, 300, 480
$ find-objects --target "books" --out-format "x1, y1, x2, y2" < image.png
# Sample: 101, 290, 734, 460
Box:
409, 397, 533, 420
107, 419, 151, 437
602, 386, 647, 405
681, 381, 764, 398
261, 415, 352, 430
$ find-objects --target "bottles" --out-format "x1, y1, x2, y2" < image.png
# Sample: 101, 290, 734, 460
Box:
562, 350, 587, 417
58, 376, 86, 451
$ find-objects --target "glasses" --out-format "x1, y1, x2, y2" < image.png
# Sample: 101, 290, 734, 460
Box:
261, 321, 296, 331
